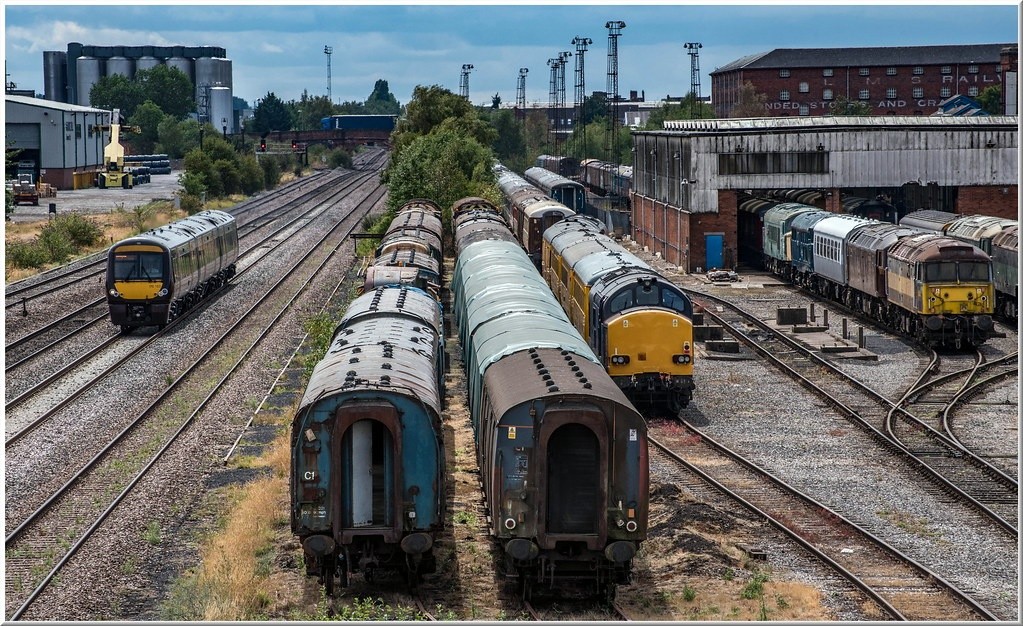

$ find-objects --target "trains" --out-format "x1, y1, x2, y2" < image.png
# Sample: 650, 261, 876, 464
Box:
523, 155, 1018, 358
486, 157, 694, 415
288, 197, 444, 589
450, 195, 649, 601
106, 209, 239, 334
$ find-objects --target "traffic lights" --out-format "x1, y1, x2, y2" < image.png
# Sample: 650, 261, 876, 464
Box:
291, 139, 296, 148
260, 139, 265, 149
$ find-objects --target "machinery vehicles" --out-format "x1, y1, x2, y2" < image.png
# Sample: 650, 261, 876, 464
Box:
13, 174, 39, 205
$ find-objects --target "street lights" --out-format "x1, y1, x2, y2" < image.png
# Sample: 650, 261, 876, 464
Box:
199, 122, 204, 151
220, 118, 228, 140
